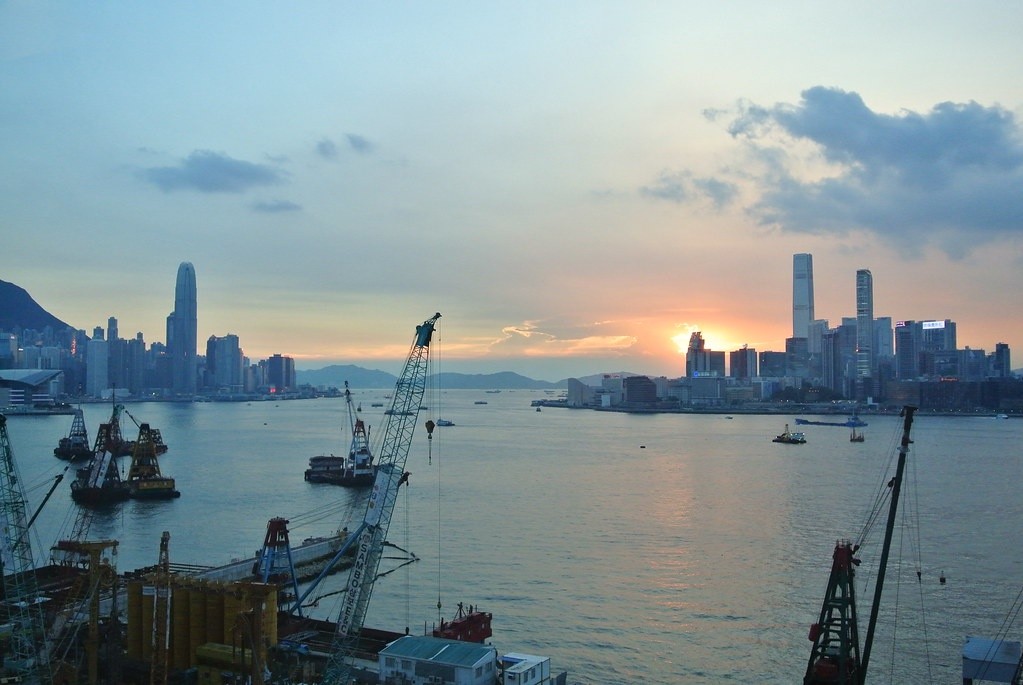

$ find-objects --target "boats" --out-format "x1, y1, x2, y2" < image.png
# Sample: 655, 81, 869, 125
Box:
849, 428, 865, 442
794, 406, 868, 428
303, 420, 411, 489
772, 423, 807, 444
106, 410, 169, 455
725, 416, 734, 420
357, 400, 363, 412
536, 407, 542, 412
68, 421, 123, 501
118, 423, 181, 497
474, 401, 488, 404
52, 405, 96, 458
486, 389, 501, 394
384, 408, 414, 416
436, 418, 455, 426
996, 413, 1009, 420
371, 403, 384, 408
419, 406, 428, 410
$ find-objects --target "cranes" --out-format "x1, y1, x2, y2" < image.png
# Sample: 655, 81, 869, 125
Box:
301, 305, 445, 685
0, 398, 140, 685
